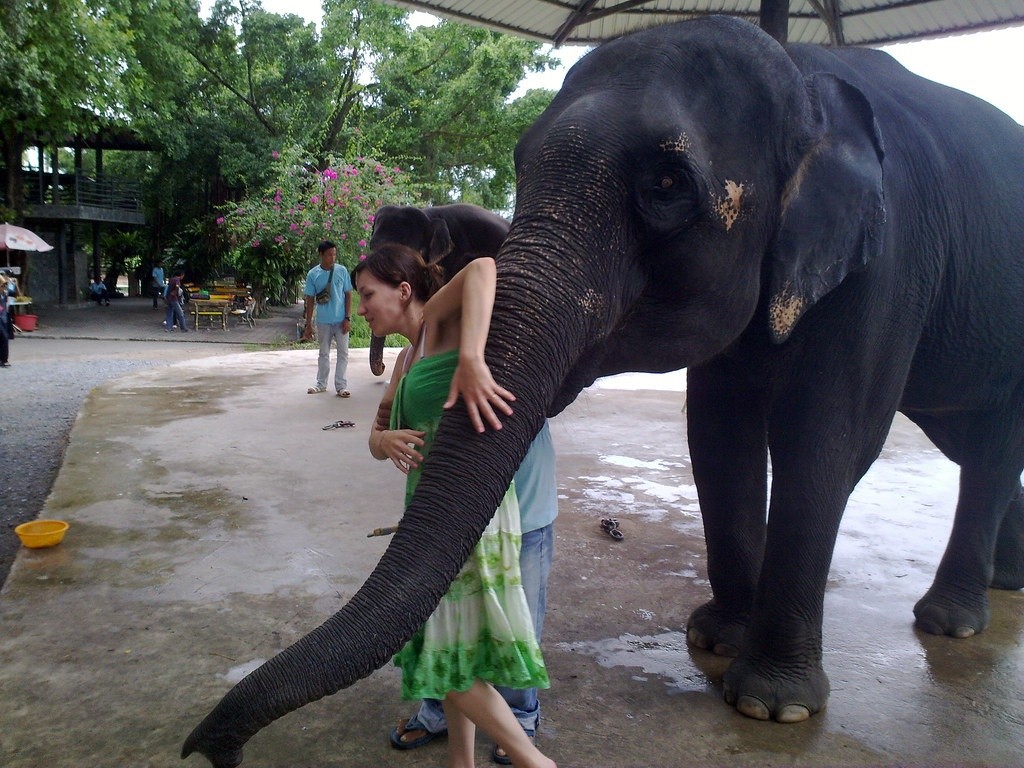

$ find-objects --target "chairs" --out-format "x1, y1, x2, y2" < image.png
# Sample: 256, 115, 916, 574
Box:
229, 300, 257, 330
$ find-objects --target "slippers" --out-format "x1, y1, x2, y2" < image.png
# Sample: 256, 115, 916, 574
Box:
307, 386, 326, 394
389, 715, 448, 749
492, 728, 536, 764
336, 389, 351, 397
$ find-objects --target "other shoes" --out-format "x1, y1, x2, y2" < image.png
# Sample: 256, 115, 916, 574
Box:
163, 321, 167, 325
173, 325, 177, 329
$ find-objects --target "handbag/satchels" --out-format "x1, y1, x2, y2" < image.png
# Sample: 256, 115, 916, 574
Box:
316, 266, 334, 304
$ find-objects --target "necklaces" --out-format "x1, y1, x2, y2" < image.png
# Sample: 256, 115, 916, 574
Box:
406, 320, 425, 374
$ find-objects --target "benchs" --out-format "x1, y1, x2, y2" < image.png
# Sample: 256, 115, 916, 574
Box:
183, 281, 252, 332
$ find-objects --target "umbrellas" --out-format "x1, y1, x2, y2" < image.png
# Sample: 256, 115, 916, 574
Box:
0, 222, 54, 252
390, 0, 1024, 49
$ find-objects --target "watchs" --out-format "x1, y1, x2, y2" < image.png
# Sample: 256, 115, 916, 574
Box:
345, 317, 351, 321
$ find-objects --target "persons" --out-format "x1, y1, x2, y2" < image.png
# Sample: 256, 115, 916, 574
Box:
0, 269, 22, 369
305, 241, 351, 398
164, 269, 192, 333
350, 245, 558, 768
152, 259, 165, 311
91, 275, 110, 306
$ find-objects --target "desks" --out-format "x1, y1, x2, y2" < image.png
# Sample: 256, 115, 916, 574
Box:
7, 301, 32, 334
216, 288, 251, 294
209, 294, 236, 306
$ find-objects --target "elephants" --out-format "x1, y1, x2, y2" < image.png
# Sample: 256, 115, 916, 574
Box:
178, 9, 1022, 768
